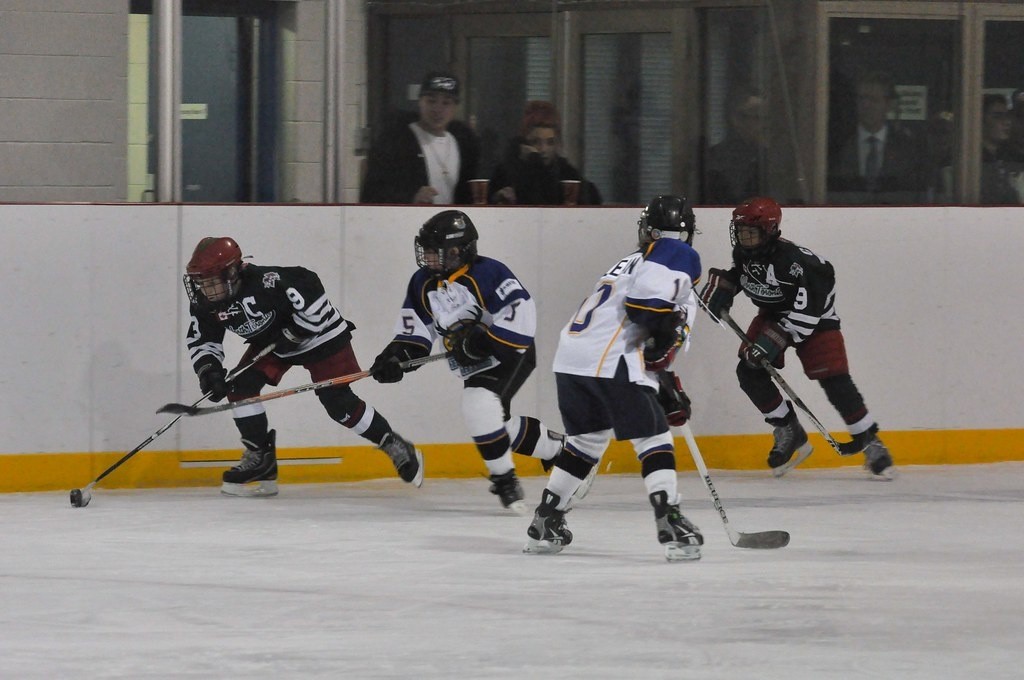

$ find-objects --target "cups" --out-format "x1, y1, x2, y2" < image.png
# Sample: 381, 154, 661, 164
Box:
468, 179, 490, 205
561, 181, 582, 206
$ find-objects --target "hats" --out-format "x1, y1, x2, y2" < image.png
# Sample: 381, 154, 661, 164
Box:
417, 71, 463, 106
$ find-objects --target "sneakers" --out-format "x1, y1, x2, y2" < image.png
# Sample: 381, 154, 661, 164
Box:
851, 422, 897, 481
522, 488, 573, 554
377, 428, 425, 488
572, 456, 601, 499
486, 468, 528, 517
220, 429, 280, 497
648, 488, 705, 562
763, 400, 814, 478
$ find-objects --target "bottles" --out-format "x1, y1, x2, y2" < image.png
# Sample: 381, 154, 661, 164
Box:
141, 173, 157, 202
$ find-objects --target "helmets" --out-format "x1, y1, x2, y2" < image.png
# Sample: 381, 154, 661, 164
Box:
413, 210, 481, 278
728, 195, 783, 249
640, 194, 697, 247
185, 237, 242, 307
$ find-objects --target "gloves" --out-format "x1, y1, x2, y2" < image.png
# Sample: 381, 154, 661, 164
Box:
658, 369, 692, 427
264, 325, 305, 359
369, 348, 413, 383
643, 323, 690, 372
194, 354, 233, 403
452, 332, 492, 367
698, 267, 743, 322
742, 324, 792, 372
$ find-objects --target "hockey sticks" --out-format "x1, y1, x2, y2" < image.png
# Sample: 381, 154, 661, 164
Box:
643, 336, 792, 551
76, 342, 277, 507
154, 349, 458, 418
720, 308, 878, 458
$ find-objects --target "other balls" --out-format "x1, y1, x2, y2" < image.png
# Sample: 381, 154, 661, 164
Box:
70, 488, 82, 508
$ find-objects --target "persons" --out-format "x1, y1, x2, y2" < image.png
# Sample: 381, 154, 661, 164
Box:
369, 72, 514, 205
699, 198, 892, 479
702, 87, 789, 200
499, 98, 602, 205
828, 73, 930, 192
369, 210, 595, 513
528, 197, 704, 558
982, 94, 1024, 205
183, 236, 424, 497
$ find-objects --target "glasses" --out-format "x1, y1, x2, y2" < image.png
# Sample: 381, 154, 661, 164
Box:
518, 136, 559, 148
